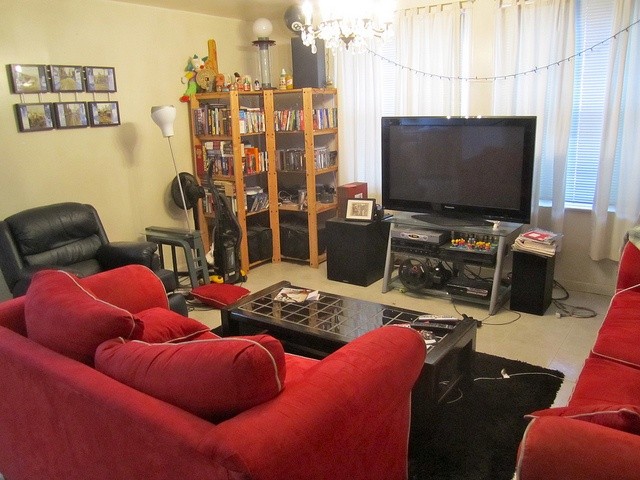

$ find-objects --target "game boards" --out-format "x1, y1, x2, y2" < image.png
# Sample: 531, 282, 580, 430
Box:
450, 236, 498, 253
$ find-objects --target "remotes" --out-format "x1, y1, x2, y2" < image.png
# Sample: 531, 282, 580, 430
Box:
411, 323, 455, 331
418, 315, 464, 321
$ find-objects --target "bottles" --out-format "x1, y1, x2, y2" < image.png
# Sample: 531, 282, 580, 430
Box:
286, 74, 293, 90
243, 77, 250, 91
279, 68, 286, 90
254, 79, 260, 91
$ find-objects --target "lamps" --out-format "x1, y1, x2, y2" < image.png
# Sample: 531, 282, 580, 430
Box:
253, 17, 277, 89
151, 104, 178, 139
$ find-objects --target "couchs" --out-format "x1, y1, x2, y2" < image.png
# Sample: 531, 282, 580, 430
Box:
2, 264, 426, 480
0, 201, 189, 320
513, 224, 640, 480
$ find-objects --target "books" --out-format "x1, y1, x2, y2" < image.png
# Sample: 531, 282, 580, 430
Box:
201, 179, 236, 214
240, 142, 268, 175
272, 286, 310, 304
511, 227, 565, 258
313, 107, 338, 130
383, 321, 439, 346
239, 106, 266, 135
274, 109, 304, 132
197, 140, 234, 177
244, 185, 269, 212
192, 104, 232, 136
275, 148, 306, 172
314, 146, 337, 169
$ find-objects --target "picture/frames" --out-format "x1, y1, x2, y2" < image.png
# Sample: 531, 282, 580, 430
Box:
16, 102, 57, 132
87, 101, 120, 127
83, 64, 117, 93
9, 64, 49, 94
344, 198, 377, 222
50, 64, 86, 93
55, 101, 90, 129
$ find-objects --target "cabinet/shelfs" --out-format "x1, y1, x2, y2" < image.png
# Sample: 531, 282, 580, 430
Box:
382, 212, 524, 318
189, 89, 282, 271
273, 86, 339, 269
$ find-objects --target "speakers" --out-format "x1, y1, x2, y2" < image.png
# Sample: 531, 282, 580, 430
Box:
291, 36, 327, 90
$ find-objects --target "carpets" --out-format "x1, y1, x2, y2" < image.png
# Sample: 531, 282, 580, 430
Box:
199, 325, 564, 480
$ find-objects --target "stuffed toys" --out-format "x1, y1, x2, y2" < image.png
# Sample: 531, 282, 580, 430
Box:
179, 52, 209, 101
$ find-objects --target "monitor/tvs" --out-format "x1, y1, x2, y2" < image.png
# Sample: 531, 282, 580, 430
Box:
381, 116, 537, 226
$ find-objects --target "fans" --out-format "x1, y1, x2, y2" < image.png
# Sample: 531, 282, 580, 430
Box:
171, 172, 215, 279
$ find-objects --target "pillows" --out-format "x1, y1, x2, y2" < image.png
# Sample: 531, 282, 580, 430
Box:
190, 283, 250, 309
525, 404, 640, 432
131, 307, 210, 344
24, 269, 144, 368
94, 334, 286, 413
